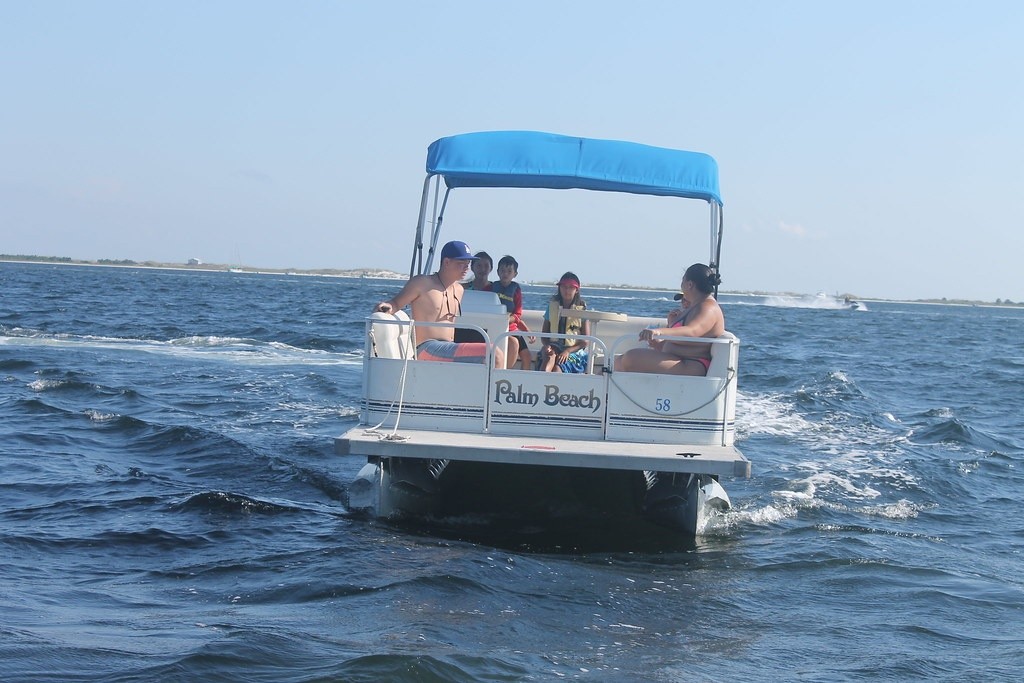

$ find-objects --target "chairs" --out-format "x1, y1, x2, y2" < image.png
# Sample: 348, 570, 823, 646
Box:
370, 309, 415, 360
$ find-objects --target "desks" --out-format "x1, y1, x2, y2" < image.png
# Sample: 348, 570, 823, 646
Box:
560, 309, 628, 374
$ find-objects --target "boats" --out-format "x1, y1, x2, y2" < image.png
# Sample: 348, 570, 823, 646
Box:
334, 131, 751, 536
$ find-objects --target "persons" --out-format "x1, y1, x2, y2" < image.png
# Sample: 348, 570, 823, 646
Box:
597, 263, 724, 375
373, 241, 536, 370
535, 272, 591, 374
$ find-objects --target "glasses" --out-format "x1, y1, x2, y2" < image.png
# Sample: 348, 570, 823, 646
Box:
445, 294, 461, 318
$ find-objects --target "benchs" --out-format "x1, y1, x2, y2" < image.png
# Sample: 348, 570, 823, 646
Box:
519, 310, 736, 377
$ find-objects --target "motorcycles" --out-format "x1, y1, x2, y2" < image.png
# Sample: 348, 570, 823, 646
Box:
839, 301, 860, 310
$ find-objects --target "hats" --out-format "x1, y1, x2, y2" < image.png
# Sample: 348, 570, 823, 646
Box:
441, 241, 480, 260
556, 278, 580, 288
